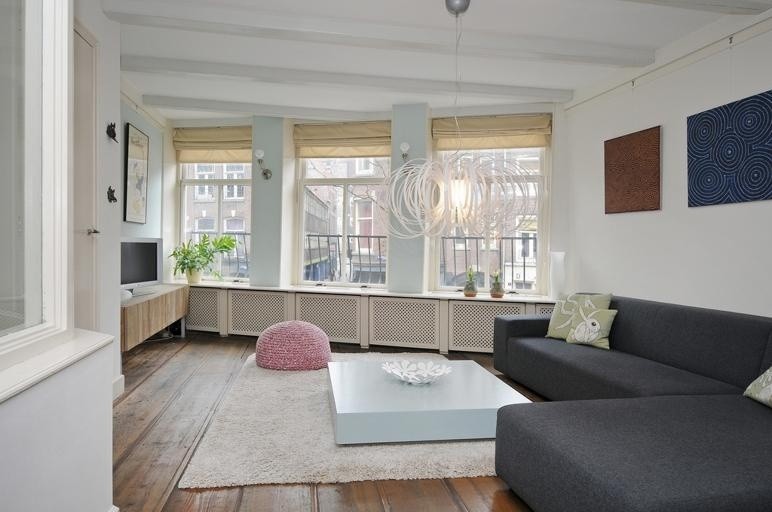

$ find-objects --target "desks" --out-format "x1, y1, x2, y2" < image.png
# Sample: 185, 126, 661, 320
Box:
120, 283, 189, 351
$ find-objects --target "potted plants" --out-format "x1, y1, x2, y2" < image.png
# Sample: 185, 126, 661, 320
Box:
464, 266, 478, 297
168, 233, 237, 282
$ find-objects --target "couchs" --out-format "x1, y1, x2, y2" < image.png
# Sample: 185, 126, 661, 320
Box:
493, 294, 771, 512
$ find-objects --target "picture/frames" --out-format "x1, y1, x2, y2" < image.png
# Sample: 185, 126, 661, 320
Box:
123, 122, 149, 224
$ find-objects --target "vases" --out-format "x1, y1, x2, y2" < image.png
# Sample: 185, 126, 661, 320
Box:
549, 251, 565, 300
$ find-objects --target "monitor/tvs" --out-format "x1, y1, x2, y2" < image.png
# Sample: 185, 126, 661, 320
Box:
118, 238, 163, 297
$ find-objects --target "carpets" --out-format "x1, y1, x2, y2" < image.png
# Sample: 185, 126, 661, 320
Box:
177, 352, 495, 489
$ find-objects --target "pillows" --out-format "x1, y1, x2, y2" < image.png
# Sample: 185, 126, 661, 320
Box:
566, 304, 618, 349
743, 366, 772, 405
546, 293, 612, 339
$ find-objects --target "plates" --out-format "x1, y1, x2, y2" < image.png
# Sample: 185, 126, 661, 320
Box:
381, 360, 455, 385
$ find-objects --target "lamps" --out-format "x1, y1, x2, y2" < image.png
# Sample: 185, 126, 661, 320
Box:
401, 142, 415, 175
255, 150, 272, 181
376, 0, 543, 240
